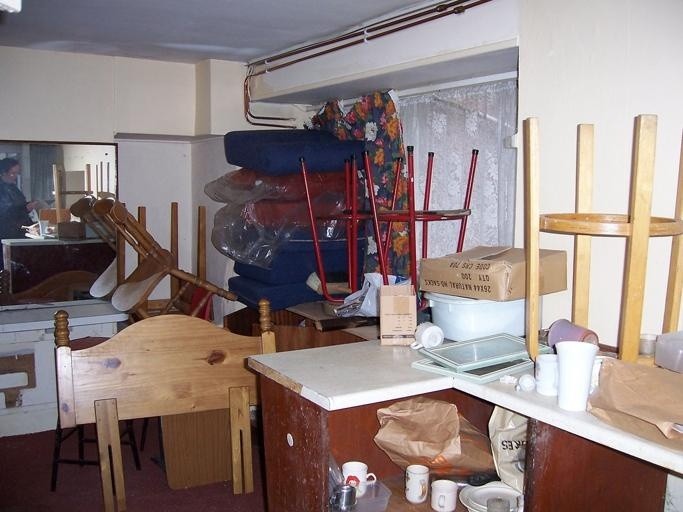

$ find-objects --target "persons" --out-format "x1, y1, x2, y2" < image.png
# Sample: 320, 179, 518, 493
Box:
1, 158, 39, 270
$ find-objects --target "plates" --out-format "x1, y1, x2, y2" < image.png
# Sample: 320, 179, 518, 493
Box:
459, 480, 523, 512
430, 480, 456, 512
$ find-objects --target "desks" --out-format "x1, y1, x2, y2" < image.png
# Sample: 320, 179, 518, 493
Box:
114, 198, 207, 326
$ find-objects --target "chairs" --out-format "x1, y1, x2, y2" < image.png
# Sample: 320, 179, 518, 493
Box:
297, 150, 403, 319
90, 196, 241, 328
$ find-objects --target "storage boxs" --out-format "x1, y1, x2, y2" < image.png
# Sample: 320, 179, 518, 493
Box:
377, 282, 418, 348
419, 285, 545, 341
415, 242, 569, 305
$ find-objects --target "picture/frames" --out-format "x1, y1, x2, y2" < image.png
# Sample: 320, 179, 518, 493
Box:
417, 332, 554, 374
410, 356, 535, 387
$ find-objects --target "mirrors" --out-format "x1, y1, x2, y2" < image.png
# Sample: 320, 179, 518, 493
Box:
0, 137, 124, 312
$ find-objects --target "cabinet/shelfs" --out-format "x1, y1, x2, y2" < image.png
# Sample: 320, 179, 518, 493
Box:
242, 337, 683, 511
0, 300, 131, 441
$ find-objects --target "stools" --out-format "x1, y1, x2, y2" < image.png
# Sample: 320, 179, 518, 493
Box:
48, 158, 112, 241
512, 107, 683, 373
46, 335, 141, 493
358, 144, 480, 317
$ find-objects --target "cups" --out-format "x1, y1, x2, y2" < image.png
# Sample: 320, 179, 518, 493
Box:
486, 497, 510, 511
546, 318, 598, 352
408, 321, 445, 352
534, 340, 617, 413
404, 463, 428, 506
338, 460, 378, 500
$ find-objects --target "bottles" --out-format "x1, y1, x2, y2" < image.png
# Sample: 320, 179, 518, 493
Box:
330, 484, 355, 511
